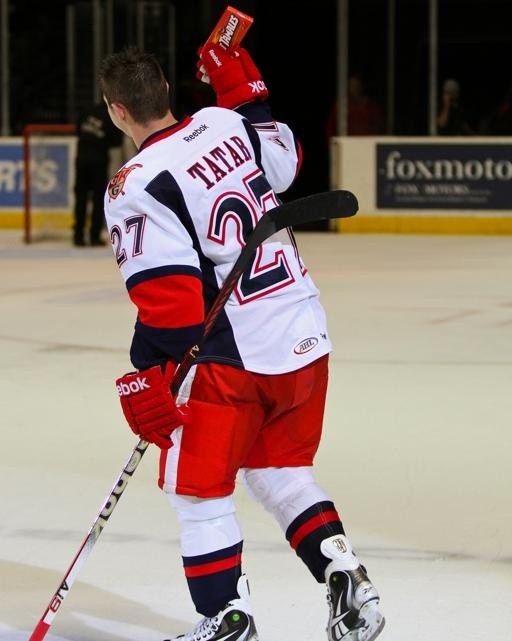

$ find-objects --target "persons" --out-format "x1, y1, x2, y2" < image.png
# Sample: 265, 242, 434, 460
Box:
435, 77, 468, 137
96, 37, 391, 641
324, 73, 387, 136
70, 92, 124, 250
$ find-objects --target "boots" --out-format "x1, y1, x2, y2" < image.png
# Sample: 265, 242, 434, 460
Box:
320, 534, 386, 641
160, 572, 260, 641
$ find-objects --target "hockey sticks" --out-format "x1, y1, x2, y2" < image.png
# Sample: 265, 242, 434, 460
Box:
29, 190, 358, 641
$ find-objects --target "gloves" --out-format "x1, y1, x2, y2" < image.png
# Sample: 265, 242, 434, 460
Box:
116, 361, 194, 449
194, 43, 269, 111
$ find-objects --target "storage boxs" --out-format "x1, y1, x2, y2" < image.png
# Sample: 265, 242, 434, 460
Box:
201, 3, 255, 58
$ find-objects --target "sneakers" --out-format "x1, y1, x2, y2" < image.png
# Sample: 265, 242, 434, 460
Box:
89, 225, 106, 247
72, 224, 86, 246
